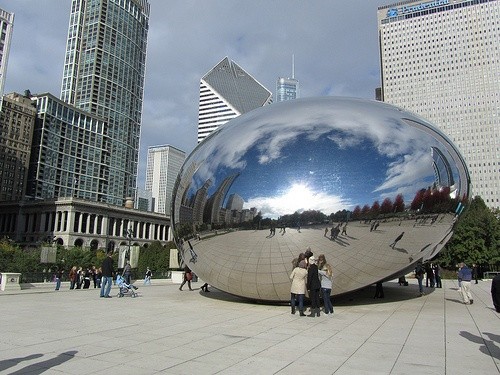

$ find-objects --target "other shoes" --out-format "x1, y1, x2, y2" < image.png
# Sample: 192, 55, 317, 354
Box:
299, 312, 306, 316
179, 286, 183, 291
470, 299, 474, 305
201, 286, 205, 291
189, 288, 194, 291
205, 290, 210, 292
417, 293, 424, 297
104, 295, 111, 298
291, 308, 296, 314
101, 295, 105, 298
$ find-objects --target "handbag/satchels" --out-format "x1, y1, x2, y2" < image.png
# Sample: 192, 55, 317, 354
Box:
186, 273, 192, 281
192, 273, 198, 282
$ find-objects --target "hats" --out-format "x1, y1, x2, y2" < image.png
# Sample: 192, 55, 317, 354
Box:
309, 256, 316, 265
305, 248, 314, 257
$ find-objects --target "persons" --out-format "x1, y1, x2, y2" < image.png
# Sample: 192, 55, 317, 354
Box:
457, 262, 479, 305
186, 222, 348, 262
359, 213, 445, 249
415, 267, 424, 296
293, 247, 327, 270
117, 273, 139, 290
143, 267, 152, 286
398, 275, 405, 286
55, 268, 64, 290
179, 266, 193, 291
68, 265, 102, 289
114, 261, 130, 285
100, 251, 116, 298
373, 282, 384, 298
289, 260, 308, 316
425, 264, 442, 290
318, 264, 334, 314
490, 275, 500, 313
307, 256, 320, 317
200, 283, 211, 292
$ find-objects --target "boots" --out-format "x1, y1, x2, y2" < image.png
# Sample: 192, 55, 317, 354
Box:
307, 307, 333, 316
